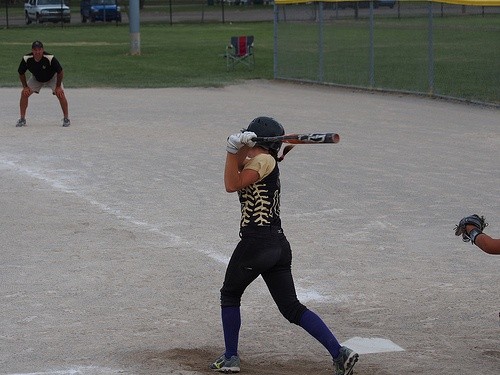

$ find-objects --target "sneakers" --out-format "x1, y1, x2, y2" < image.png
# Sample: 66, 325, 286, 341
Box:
16, 118, 26, 127
63, 118, 70, 127
209, 351, 241, 373
332, 346, 359, 375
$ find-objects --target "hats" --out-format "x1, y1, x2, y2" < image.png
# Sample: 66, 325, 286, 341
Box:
32, 41, 43, 49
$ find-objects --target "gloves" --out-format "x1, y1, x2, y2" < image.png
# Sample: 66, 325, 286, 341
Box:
226, 131, 257, 154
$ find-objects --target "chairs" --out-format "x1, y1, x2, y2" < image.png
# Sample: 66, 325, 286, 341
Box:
224, 35, 255, 69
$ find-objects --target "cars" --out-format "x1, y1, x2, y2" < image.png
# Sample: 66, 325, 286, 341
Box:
81, 0, 121, 23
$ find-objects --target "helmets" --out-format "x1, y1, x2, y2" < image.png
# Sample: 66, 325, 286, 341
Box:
241, 117, 285, 153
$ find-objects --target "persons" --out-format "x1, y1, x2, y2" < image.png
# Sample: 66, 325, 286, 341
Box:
454, 214, 500, 257
208, 117, 360, 375
16, 41, 70, 127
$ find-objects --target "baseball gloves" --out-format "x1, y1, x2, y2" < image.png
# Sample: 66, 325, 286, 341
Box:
454, 213, 488, 243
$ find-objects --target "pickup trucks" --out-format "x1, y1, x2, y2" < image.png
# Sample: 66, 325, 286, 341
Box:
25, 0, 70, 25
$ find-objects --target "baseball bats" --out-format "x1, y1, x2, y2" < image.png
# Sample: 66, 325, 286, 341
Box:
226, 133, 341, 145
